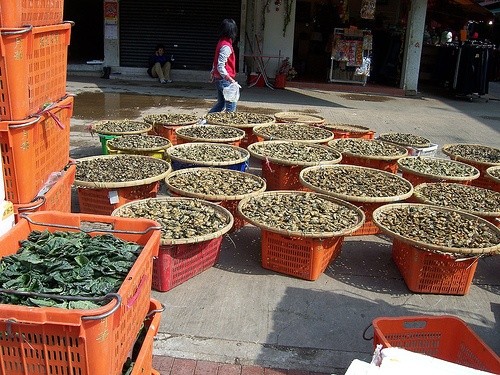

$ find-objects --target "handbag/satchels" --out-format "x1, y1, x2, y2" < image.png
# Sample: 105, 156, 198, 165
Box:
223, 81, 240, 102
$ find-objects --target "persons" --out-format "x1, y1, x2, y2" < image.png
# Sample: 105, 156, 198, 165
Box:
147, 46, 173, 84
208, 19, 237, 113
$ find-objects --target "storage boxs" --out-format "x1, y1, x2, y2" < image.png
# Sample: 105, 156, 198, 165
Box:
0, 0, 500, 375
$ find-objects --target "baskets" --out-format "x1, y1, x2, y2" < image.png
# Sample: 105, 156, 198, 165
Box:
0, 210, 165, 375
362, 315, 500, 374
0, 0, 78, 214
72, 111, 500, 296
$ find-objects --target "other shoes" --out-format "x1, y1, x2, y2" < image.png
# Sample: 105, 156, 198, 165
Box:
160, 79, 167, 83
165, 79, 173, 83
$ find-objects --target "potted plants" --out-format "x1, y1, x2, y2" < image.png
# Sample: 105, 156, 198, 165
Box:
275, 57, 296, 89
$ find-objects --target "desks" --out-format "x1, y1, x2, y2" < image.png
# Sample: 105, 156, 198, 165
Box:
243, 55, 283, 90
